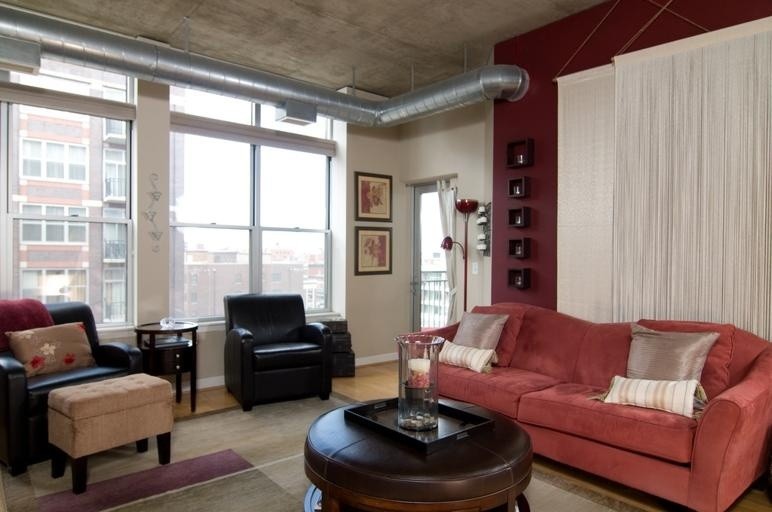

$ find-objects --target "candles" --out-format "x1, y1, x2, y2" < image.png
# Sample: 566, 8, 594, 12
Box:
407, 357, 432, 390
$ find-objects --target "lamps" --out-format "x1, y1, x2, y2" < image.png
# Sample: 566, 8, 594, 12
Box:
440, 198, 478, 312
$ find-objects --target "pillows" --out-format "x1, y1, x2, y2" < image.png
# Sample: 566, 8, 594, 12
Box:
5, 321, 98, 378
436, 340, 498, 373
0, 298, 54, 353
626, 322, 721, 380
588, 376, 708, 419
452, 313, 509, 350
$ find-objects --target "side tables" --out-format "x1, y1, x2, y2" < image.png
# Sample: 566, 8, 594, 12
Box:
134, 321, 199, 413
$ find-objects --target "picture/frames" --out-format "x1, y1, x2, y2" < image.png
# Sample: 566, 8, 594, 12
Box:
354, 226, 393, 276
354, 171, 392, 223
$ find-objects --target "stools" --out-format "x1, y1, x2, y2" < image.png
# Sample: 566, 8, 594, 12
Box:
49, 373, 174, 493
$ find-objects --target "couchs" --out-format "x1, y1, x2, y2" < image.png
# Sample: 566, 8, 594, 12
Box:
0, 301, 148, 476
223, 294, 334, 413
396, 302, 772, 512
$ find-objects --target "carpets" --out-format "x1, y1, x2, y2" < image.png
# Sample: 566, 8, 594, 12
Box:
0, 391, 649, 512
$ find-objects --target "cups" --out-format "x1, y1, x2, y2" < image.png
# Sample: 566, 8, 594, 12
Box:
160, 318, 177, 330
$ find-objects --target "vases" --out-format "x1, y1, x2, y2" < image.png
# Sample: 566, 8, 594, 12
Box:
393, 335, 445, 430
